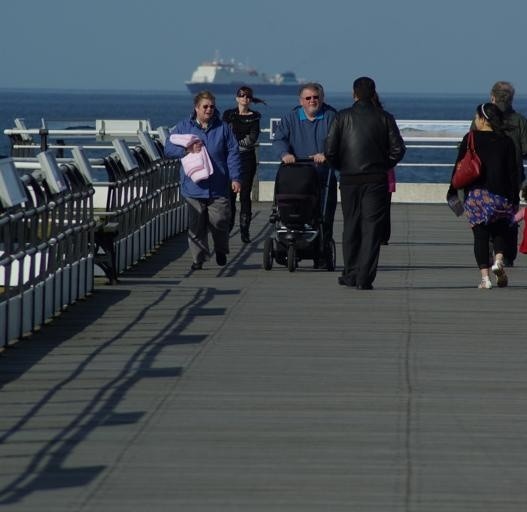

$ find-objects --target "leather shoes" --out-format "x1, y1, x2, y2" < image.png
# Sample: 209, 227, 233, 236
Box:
242, 236, 251, 242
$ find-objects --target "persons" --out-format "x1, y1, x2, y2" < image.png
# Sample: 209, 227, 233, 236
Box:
273, 77, 406, 290
164, 90, 242, 270
448, 103, 520, 289
512, 185, 527, 254
470, 81, 527, 267
221, 86, 266, 242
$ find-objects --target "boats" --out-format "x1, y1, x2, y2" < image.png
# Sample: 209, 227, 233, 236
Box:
183, 47, 312, 95
0, 115, 171, 324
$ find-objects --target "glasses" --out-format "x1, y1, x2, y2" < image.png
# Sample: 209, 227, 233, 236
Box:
492, 261, 507, 287
203, 105, 214, 109
239, 93, 250, 98
303, 96, 319, 100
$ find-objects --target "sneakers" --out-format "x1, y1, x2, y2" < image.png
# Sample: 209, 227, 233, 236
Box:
192, 263, 202, 270
478, 278, 492, 288
216, 254, 227, 266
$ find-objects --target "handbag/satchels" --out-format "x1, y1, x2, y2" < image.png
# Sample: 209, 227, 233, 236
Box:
452, 150, 482, 188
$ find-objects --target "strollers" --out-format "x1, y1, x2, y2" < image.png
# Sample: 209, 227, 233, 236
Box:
263, 157, 336, 273
